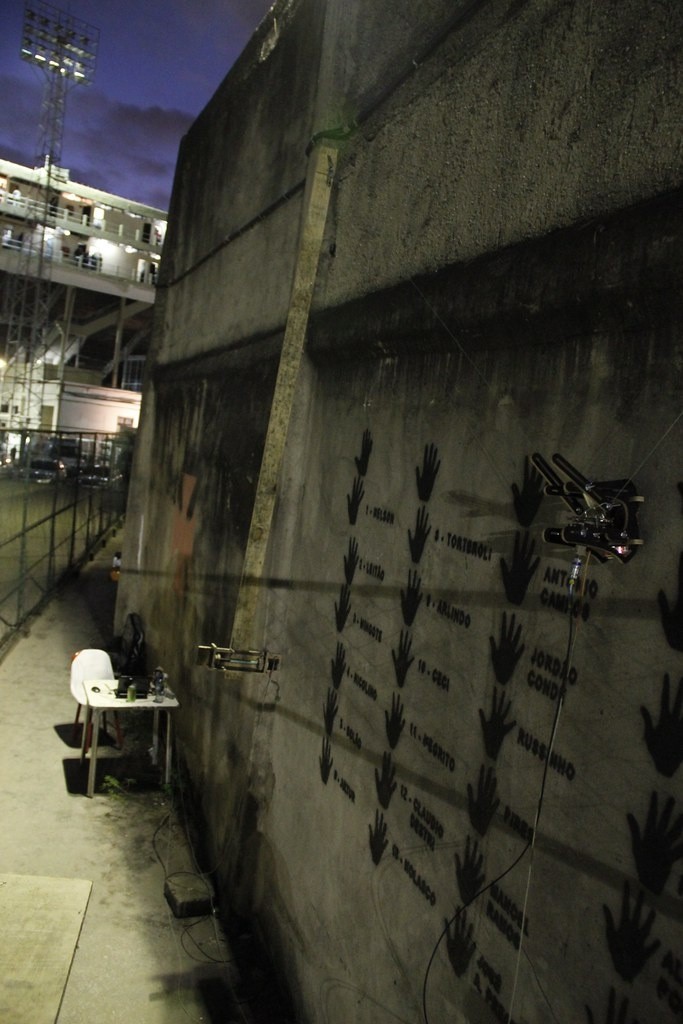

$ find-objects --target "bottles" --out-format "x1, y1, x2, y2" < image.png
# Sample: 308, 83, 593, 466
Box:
153, 670, 165, 703
127, 678, 137, 702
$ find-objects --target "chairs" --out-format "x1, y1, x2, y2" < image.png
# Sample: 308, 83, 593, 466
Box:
70, 649, 124, 754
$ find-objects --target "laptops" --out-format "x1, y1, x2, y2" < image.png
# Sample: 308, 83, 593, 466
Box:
114, 674, 152, 698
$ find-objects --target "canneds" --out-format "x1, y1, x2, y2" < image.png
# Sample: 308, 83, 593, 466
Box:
127, 686, 136, 702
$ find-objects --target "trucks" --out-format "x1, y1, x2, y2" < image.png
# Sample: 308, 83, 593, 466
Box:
48, 438, 81, 477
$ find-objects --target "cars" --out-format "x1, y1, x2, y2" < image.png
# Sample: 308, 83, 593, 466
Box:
17, 457, 67, 485
78, 464, 121, 487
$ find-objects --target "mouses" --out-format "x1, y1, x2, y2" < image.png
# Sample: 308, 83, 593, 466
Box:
92, 686, 100, 693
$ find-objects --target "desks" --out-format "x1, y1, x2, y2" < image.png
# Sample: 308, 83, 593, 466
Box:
80, 680, 179, 798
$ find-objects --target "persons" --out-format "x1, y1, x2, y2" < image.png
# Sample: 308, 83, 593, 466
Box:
110, 552, 122, 586
